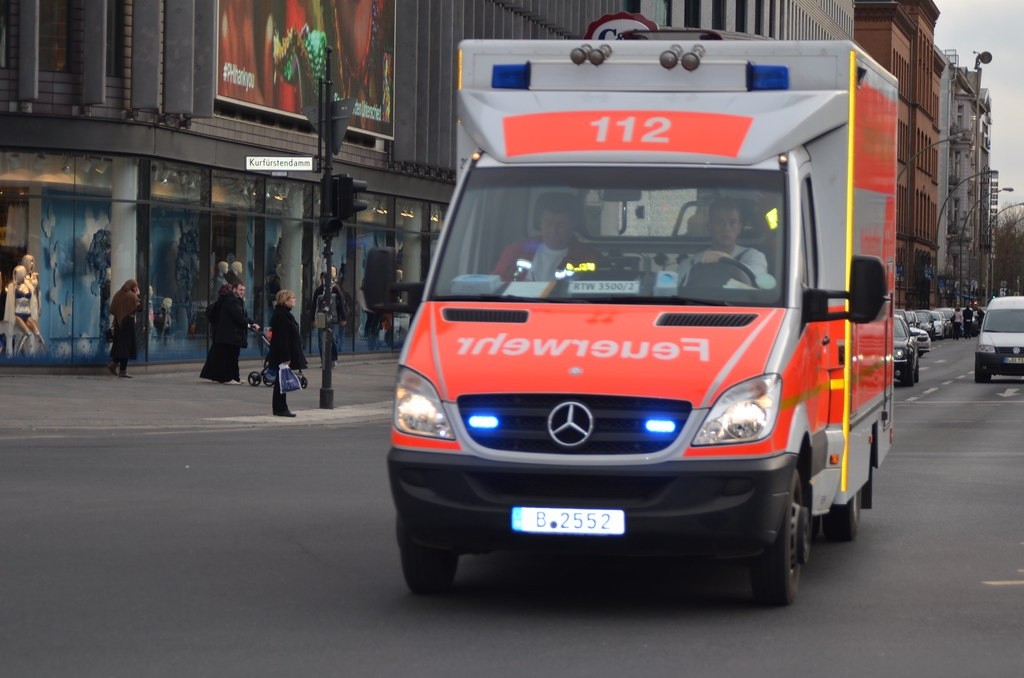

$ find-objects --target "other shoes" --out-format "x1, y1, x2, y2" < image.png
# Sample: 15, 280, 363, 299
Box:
278, 411, 296, 417
223, 380, 240, 385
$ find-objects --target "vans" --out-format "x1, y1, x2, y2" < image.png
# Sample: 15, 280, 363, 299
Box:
975, 296, 1024, 384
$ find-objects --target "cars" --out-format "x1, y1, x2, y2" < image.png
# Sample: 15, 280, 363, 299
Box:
909, 327, 931, 358
893, 307, 986, 342
892, 314, 920, 387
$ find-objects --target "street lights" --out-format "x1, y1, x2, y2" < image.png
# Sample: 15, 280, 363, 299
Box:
980, 202, 1024, 307
934, 170, 998, 309
959, 187, 1014, 308
897, 133, 964, 181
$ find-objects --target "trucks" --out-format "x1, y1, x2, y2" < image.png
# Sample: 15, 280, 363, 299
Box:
358, 27, 898, 611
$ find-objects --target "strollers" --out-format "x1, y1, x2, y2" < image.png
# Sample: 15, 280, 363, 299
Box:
247, 323, 308, 390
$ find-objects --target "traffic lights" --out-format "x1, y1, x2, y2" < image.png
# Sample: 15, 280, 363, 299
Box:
332, 173, 368, 219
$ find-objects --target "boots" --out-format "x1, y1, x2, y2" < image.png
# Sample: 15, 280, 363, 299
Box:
107, 361, 118, 377
118, 368, 133, 378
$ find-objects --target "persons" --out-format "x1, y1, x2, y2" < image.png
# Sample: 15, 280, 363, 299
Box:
108, 279, 143, 379
264, 289, 308, 418
214, 261, 248, 303
396, 270, 402, 282
952, 304, 973, 340
331, 266, 336, 282
664, 195, 768, 292
4, 254, 47, 359
149, 284, 172, 347
200, 283, 260, 384
309, 271, 347, 369
493, 192, 610, 281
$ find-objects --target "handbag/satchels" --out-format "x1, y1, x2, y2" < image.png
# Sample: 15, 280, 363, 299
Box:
950, 313, 955, 323
205, 302, 220, 325
106, 328, 114, 343
279, 363, 302, 393
